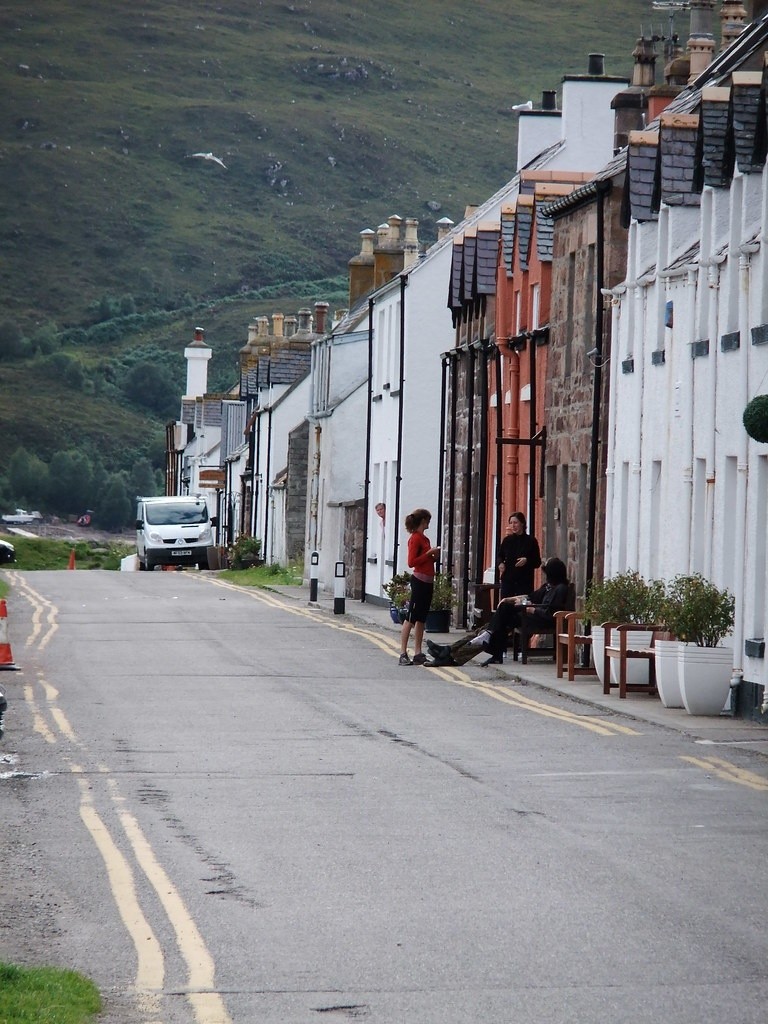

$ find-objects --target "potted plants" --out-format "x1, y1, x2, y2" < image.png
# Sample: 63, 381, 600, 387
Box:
236, 536, 265, 570
393, 589, 412, 623
580, 568, 639, 684
668, 570, 736, 716
654, 592, 705, 708
610, 577, 666, 683
381, 571, 412, 623
425, 572, 465, 633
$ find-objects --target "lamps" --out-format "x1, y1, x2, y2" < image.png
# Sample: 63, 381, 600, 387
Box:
471, 340, 485, 350
460, 344, 473, 353
439, 351, 451, 360
449, 348, 460, 356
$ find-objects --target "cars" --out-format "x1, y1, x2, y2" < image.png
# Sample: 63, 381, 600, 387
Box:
0, 538, 18, 565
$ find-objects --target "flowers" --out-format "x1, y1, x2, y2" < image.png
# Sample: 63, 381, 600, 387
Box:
401, 599, 411, 610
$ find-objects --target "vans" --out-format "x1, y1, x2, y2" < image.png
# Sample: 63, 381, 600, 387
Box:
135, 495, 223, 570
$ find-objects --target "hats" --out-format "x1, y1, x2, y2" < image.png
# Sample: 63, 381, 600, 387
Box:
541, 557, 567, 572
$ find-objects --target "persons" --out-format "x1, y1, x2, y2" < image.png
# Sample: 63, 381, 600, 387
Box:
375, 503, 386, 540
480, 557, 573, 667
423, 557, 568, 667
496, 512, 542, 660
399, 509, 440, 666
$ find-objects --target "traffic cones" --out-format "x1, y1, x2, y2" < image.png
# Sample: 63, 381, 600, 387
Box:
65, 547, 77, 570
0, 597, 21, 672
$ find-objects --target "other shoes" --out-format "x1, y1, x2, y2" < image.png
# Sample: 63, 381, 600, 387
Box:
412, 653, 430, 665
426, 640, 451, 653
482, 640, 494, 655
423, 656, 453, 666
482, 656, 503, 667
399, 652, 414, 666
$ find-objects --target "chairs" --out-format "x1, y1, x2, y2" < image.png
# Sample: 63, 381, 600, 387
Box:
553, 599, 627, 681
511, 583, 575, 664
470, 582, 502, 619
600, 601, 681, 699
